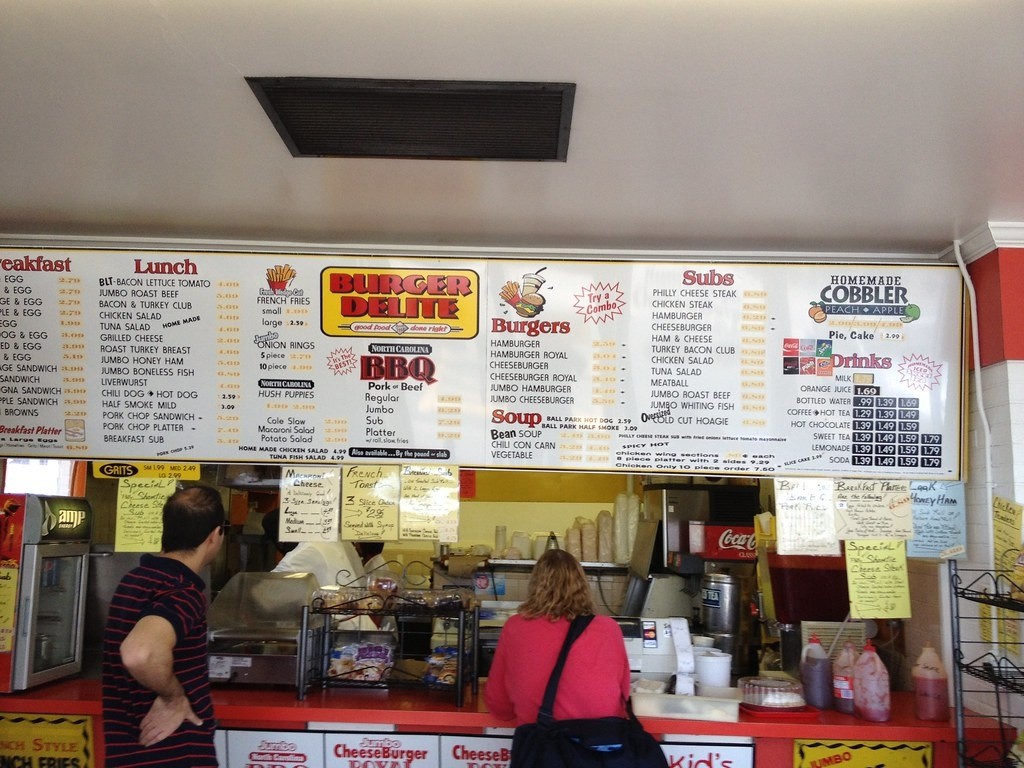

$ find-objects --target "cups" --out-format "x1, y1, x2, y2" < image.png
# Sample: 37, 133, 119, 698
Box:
693, 636, 714, 647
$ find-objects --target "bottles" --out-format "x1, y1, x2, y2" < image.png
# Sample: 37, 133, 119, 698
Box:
1011, 543, 1024, 603
833, 636, 860, 715
800, 633, 833, 711
912, 641, 949, 721
854, 639, 890, 722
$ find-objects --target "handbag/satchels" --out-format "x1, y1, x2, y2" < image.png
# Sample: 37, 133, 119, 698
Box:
509, 717, 669, 768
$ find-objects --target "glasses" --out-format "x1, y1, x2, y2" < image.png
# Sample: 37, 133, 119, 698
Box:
219, 520, 231, 534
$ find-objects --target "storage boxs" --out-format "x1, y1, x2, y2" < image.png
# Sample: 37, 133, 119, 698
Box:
628, 683, 744, 724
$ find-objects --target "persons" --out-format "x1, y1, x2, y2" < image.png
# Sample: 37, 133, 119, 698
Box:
262, 507, 365, 630
484, 548, 630, 725
102, 486, 226, 768
351, 542, 396, 630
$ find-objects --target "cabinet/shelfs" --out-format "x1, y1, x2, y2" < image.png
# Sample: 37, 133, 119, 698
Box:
948, 558, 1024, 768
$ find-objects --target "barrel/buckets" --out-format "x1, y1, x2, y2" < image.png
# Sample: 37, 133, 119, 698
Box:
696, 651, 732, 686
699, 562, 756, 687
691, 646, 721, 672
